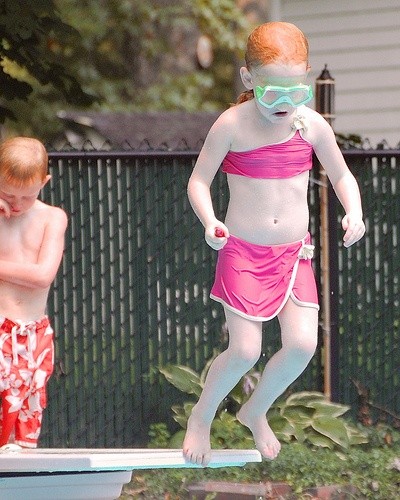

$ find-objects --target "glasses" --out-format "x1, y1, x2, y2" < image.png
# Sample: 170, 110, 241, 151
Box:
250, 77, 314, 108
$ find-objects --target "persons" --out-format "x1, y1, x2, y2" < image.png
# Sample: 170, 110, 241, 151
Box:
0, 137, 68, 450
183, 21, 366, 466
